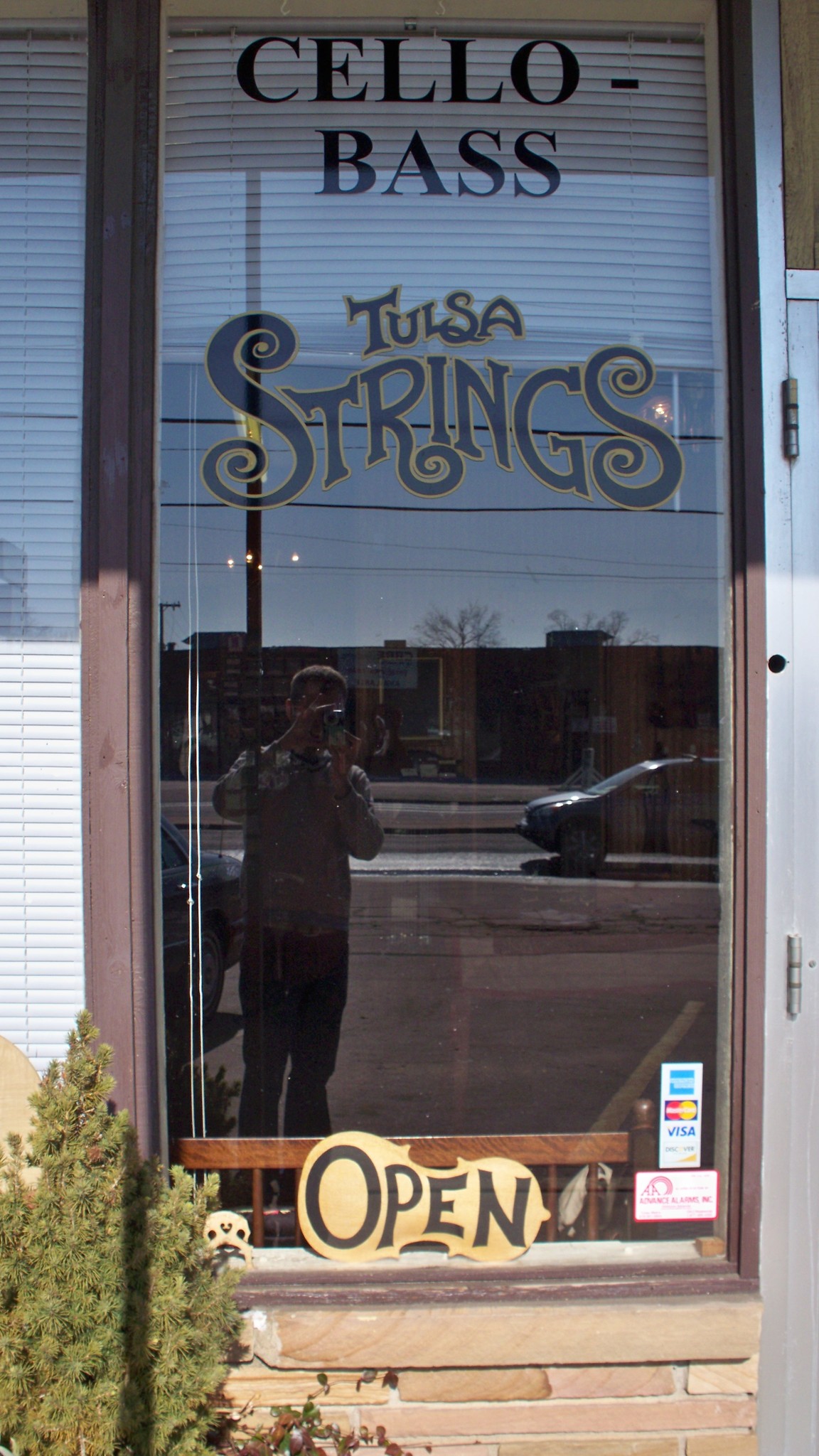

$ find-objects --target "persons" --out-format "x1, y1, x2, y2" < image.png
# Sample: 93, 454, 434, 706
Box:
212, 664, 383, 1210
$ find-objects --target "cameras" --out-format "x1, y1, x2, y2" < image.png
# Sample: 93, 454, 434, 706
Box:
318, 708, 347, 734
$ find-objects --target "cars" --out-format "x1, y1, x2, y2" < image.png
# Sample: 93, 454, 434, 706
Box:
160, 817, 248, 1021
515, 754, 720, 868
